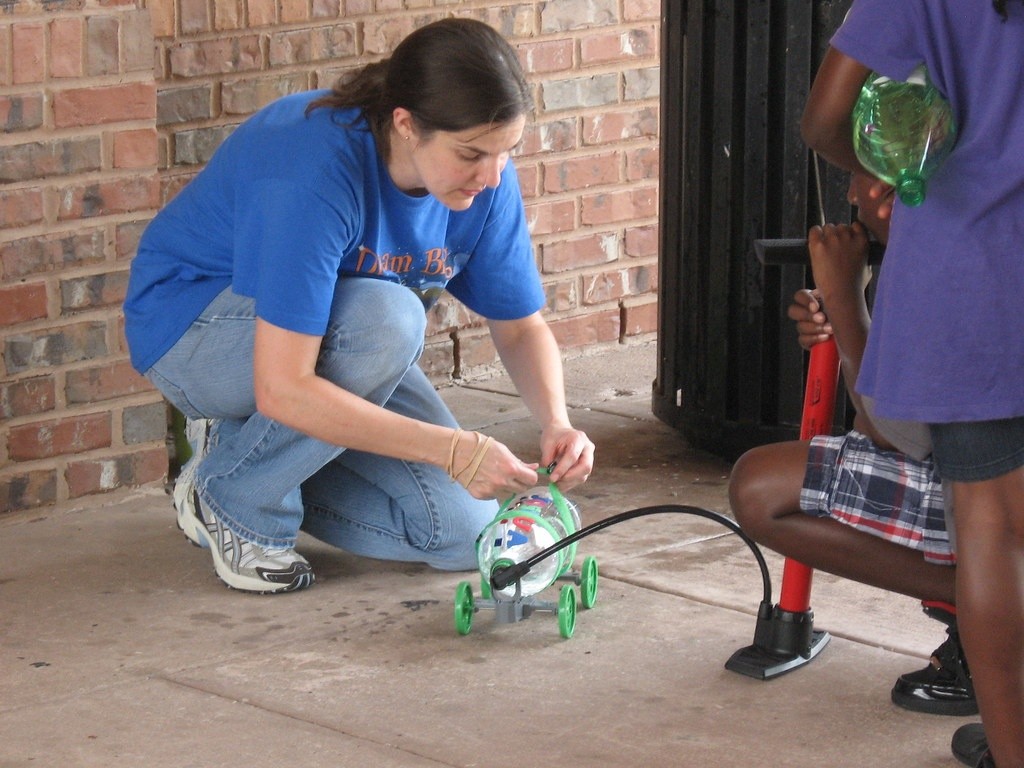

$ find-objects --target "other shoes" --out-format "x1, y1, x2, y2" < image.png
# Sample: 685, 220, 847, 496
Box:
951, 723, 995, 768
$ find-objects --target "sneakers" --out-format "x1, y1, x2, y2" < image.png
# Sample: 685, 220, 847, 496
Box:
891, 600, 980, 716
173, 416, 219, 510
177, 480, 315, 595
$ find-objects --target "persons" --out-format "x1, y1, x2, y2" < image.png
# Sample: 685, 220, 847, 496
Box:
729, 0, 1024, 768
123, 19, 596, 593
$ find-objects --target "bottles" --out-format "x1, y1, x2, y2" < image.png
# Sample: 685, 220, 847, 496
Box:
851, 65, 958, 210
477, 487, 583, 598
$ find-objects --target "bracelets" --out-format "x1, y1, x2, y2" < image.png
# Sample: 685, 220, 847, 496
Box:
446, 428, 494, 490
